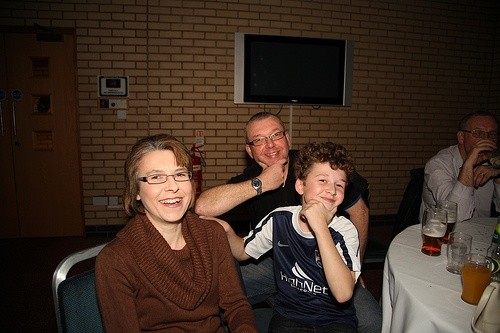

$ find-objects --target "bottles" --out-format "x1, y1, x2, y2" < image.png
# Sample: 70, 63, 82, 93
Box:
484, 214, 500, 283
482, 150, 500, 169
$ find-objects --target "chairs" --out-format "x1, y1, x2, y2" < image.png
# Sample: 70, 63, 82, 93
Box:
53, 242, 247, 333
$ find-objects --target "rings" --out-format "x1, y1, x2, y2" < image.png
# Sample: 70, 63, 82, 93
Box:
480, 172, 485, 177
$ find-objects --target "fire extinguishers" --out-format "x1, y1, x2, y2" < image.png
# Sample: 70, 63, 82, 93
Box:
190, 144, 206, 193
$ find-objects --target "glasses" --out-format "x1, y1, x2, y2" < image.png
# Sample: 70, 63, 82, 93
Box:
141, 172, 192, 184
249, 131, 286, 146
461, 129, 500, 139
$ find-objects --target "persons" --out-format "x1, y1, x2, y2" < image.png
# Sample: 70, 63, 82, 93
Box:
200, 140, 361, 333
419, 110, 500, 226
95, 133, 256, 333
195, 113, 383, 333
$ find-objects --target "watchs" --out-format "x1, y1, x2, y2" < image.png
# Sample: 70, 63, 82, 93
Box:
250, 178, 262, 195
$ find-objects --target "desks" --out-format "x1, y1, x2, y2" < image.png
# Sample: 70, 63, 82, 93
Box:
378, 216, 500, 333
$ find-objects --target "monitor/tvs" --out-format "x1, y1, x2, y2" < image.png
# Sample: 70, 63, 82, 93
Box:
234, 32, 353, 107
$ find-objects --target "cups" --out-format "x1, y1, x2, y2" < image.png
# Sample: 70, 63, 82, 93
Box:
460, 254, 492, 306
445, 232, 472, 275
435, 200, 458, 244
421, 207, 448, 256
